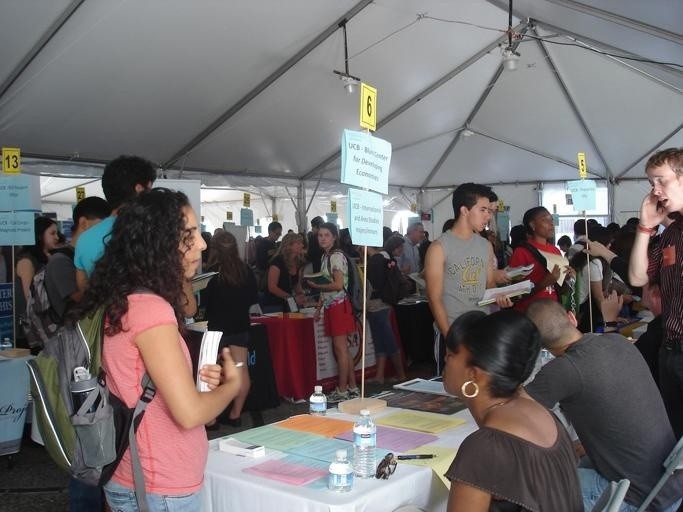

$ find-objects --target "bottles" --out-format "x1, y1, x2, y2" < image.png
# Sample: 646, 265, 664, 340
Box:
539, 347, 550, 360
0, 337, 12, 352
69, 365, 104, 414
307, 385, 327, 416
324, 447, 354, 492
352, 408, 376, 480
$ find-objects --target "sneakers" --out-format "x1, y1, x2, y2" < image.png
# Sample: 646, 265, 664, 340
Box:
205, 422, 219, 431
219, 417, 241, 427
347, 384, 361, 399
326, 386, 349, 403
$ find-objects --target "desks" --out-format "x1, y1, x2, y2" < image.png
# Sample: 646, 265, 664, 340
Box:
204, 336, 638, 510
1, 294, 433, 456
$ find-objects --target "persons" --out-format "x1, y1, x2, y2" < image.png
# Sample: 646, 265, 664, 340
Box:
524, 300, 682, 512
66, 187, 240, 512
600, 277, 666, 389
73, 155, 196, 325
199, 217, 455, 431
442, 309, 585, 512
2, 196, 111, 353
629, 147, 682, 440
425, 183, 513, 364
476, 206, 659, 332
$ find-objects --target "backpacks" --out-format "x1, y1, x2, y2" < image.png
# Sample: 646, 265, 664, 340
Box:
22, 248, 74, 353
321, 250, 372, 311
560, 256, 596, 318
368, 248, 408, 305
25, 287, 157, 512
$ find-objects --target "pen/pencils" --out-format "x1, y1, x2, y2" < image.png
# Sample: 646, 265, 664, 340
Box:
398, 454, 439, 460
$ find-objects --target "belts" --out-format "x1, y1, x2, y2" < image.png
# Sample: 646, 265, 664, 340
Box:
662, 338, 683, 352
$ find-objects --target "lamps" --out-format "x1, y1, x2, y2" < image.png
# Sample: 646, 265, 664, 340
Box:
344, 78, 358, 96
503, 49, 519, 70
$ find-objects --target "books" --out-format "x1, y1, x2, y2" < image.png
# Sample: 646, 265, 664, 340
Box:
183, 329, 223, 391
192, 271, 219, 296
511, 270, 533, 277
